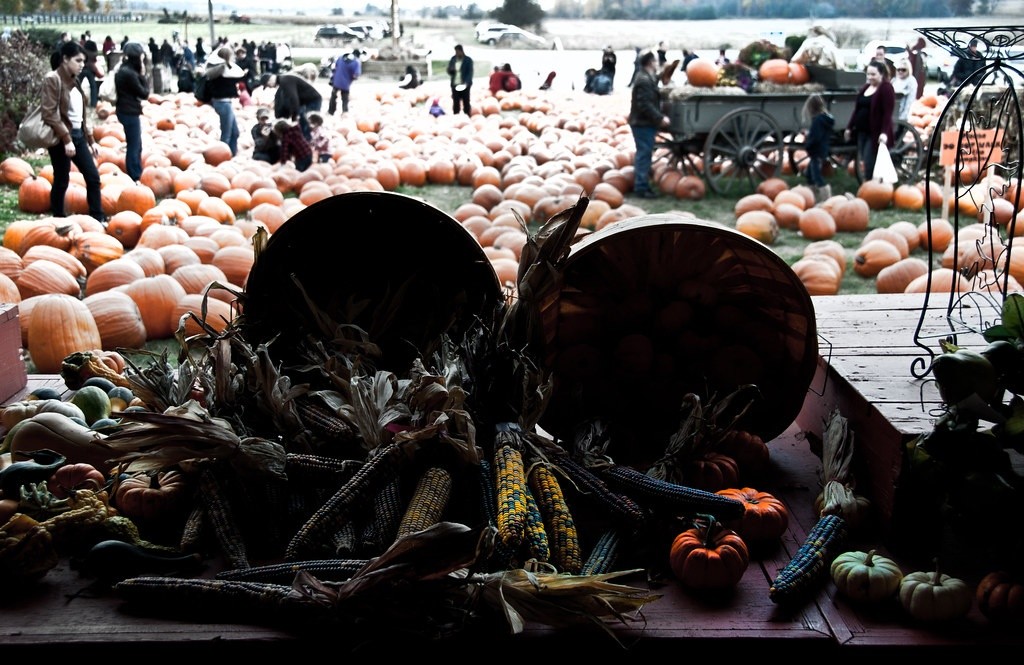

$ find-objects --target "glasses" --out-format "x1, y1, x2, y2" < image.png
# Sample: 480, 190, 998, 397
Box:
897, 68, 906, 71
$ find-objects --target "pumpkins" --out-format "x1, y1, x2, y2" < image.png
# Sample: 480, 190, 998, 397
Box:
0, 350, 191, 598
1, 87, 636, 375
670, 430, 789, 589
829, 548, 972, 623
976, 561, 1024, 621
648, 57, 1024, 295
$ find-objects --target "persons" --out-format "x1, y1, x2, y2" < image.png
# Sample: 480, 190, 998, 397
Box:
398, 66, 424, 89
148, 32, 332, 157
489, 64, 522, 96
327, 49, 362, 115
627, 49, 671, 199
582, 42, 730, 95
844, 61, 895, 182
56, 30, 153, 186
42, 42, 110, 222
251, 72, 332, 172
791, 26, 851, 71
950, 40, 986, 87
863, 38, 927, 176
447, 45, 473, 118
800, 93, 836, 208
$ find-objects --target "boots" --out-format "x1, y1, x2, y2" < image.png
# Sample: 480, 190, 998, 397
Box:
805, 184, 817, 195
817, 184, 831, 203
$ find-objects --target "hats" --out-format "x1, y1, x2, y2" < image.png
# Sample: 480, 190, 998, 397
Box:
971, 40, 977, 46
661, 60, 680, 85
257, 107, 270, 120
123, 43, 144, 58
260, 73, 272, 91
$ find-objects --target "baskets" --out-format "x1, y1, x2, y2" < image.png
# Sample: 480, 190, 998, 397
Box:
242, 191, 508, 380
541, 210, 833, 444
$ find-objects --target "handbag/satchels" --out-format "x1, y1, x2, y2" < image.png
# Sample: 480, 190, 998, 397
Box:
872, 142, 898, 184
18, 71, 62, 148
194, 68, 210, 102
98, 61, 122, 106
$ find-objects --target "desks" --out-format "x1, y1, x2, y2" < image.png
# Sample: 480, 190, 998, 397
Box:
757, 293, 1024, 665
0, 373, 837, 665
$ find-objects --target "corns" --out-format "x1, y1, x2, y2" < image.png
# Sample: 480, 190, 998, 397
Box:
88, 398, 748, 620
769, 514, 848, 602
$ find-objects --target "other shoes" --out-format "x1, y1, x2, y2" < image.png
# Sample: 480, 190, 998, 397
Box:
633, 190, 659, 198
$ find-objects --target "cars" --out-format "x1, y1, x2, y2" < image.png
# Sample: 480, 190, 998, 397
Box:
860, 39, 1024, 91
313, 19, 392, 45
474, 22, 544, 47
